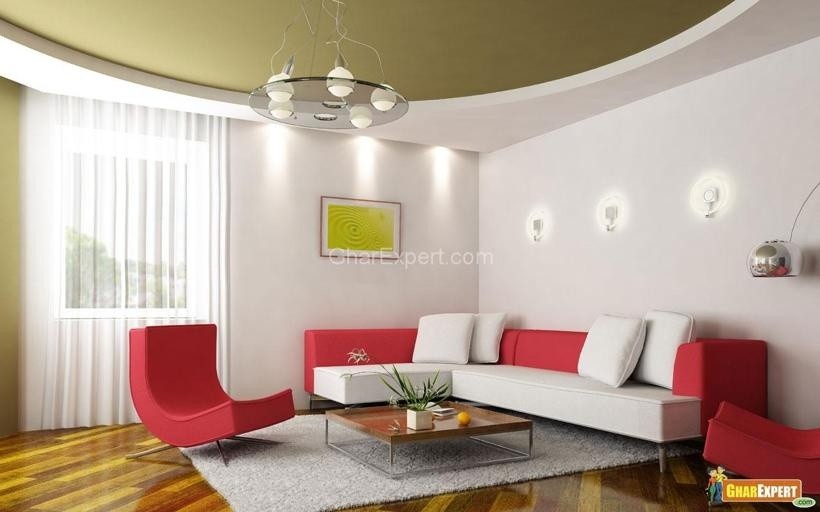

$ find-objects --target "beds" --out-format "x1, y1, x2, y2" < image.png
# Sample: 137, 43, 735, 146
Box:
325, 400, 534, 479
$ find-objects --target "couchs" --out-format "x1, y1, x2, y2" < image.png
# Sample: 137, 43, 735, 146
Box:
304, 328, 768, 473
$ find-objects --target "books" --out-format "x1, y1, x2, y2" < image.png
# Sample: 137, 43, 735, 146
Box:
407, 402, 457, 417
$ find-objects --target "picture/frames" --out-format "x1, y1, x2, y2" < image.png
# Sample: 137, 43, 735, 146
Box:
320, 196, 401, 259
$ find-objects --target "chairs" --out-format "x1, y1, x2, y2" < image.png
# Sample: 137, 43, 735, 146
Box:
700, 396, 819, 512
126, 324, 295, 467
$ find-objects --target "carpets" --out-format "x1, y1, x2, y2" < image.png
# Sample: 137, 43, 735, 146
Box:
174, 401, 704, 512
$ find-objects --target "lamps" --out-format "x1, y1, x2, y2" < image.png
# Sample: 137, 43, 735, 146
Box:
249, 0, 408, 129
748, 176, 820, 278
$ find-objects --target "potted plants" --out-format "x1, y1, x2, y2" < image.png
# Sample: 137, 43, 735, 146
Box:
340, 348, 450, 431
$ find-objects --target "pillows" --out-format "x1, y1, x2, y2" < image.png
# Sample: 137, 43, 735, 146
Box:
577, 315, 647, 387
412, 313, 475, 365
469, 313, 508, 363
633, 310, 693, 390
